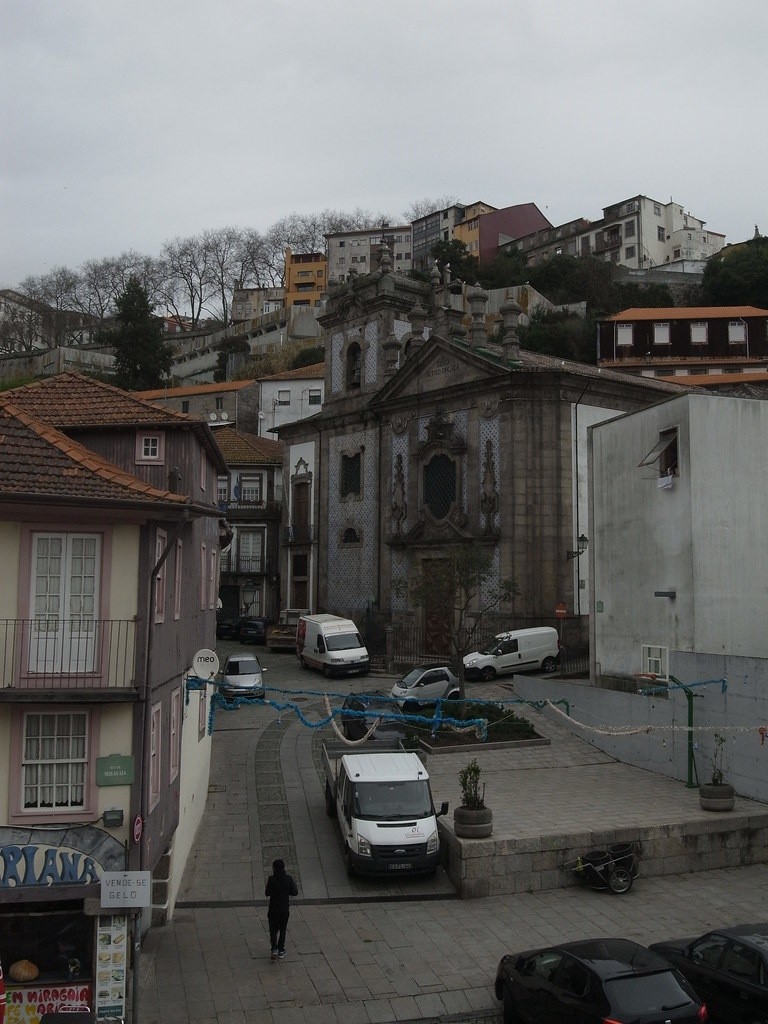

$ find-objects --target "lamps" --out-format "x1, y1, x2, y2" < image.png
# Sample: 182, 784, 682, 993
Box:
101, 809, 125, 827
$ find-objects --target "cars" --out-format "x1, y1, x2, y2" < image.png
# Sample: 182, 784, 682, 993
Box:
217, 654, 268, 701
495, 937, 709, 1024
389, 662, 467, 711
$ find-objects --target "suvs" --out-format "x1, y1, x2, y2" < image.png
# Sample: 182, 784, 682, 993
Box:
238, 616, 276, 646
647, 923, 768, 1024
341, 690, 421, 755
215, 614, 245, 641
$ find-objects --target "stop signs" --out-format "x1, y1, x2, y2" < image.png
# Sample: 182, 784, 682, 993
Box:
554, 603, 568, 618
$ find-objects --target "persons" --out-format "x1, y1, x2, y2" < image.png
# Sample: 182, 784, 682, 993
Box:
265, 859, 298, 960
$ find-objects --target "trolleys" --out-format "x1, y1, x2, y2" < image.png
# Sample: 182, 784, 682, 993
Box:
563, 852, 641, 894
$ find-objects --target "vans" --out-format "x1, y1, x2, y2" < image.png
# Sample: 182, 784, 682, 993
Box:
461, 627, 563, 680
296, 613, 371, 679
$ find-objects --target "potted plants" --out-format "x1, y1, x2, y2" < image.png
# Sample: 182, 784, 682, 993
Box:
700, 734, 735, 812
453, 756, 494, 838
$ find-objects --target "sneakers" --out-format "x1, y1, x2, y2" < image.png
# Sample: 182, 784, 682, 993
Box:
278, 950, 288, 959
271, 950, 278, 960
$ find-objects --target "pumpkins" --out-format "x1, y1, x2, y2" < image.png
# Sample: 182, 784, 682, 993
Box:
9, 959, 38, 982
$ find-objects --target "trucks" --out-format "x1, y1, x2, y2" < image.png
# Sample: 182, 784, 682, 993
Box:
320, 737, 450, 879
